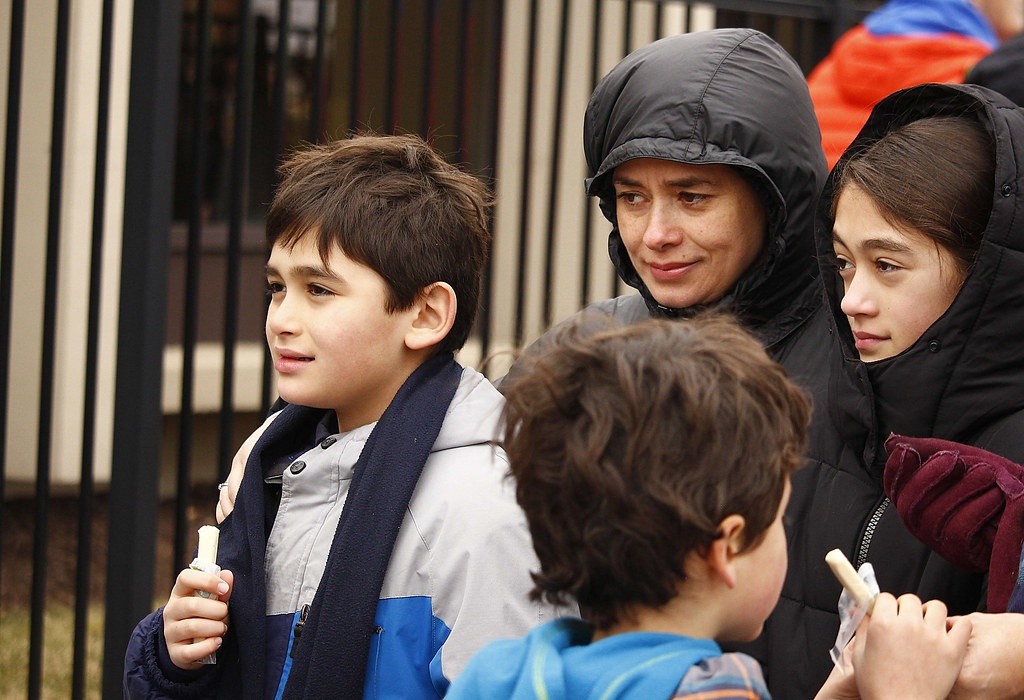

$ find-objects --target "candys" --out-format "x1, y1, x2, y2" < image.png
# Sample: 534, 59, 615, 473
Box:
195, 523, 218, 576
824, 548, 876, 613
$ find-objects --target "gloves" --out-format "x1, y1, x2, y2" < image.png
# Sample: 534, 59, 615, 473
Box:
882, 431, 1024, 614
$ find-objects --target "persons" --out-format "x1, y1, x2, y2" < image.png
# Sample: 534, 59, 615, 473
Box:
576, 27, 845, 700
732, 81, 1024, 698
795, 0, 1023, 182
275, 56, 317, 144
425, 293, 975, 700
118, 129, 591, 700
